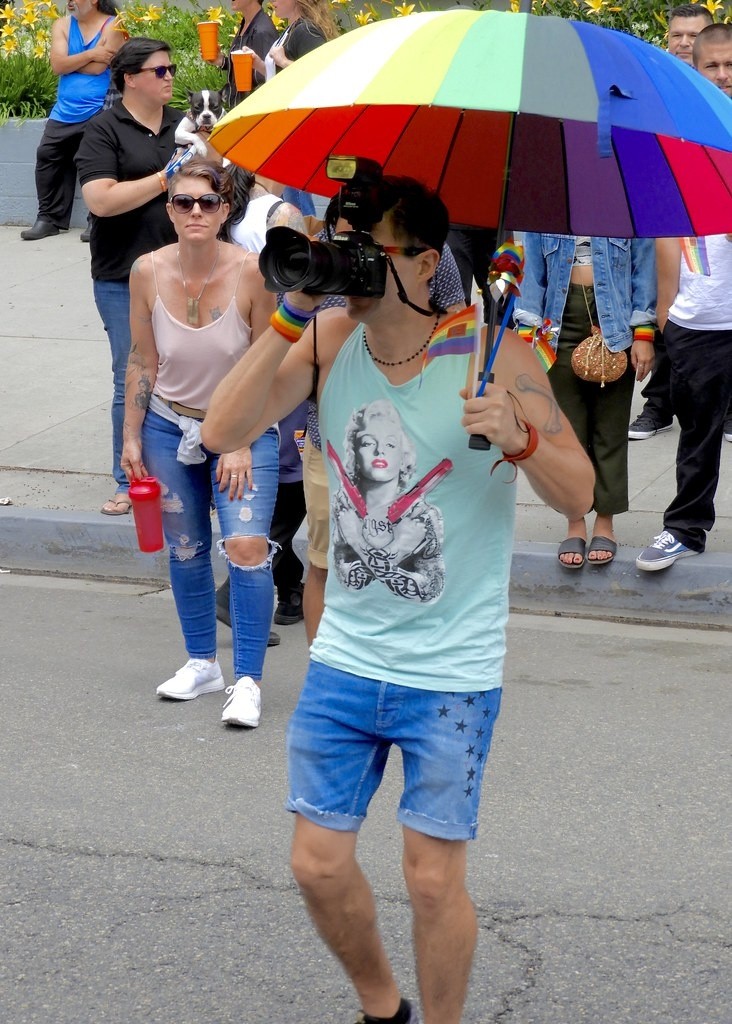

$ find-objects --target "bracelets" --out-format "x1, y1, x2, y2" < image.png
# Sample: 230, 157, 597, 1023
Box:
271, 298, 319, 342
502, 421, 538, 461
634, 325, 655, 341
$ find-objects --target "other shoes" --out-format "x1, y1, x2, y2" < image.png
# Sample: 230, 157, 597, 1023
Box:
214, 577, 280, 645
273, 583, 304, 624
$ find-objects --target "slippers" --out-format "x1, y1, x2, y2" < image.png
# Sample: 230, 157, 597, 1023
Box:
99, 499, 133, 516
557, 536, 585, 569
587, 535, 617, 564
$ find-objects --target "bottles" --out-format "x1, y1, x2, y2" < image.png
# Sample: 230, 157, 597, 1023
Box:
128, 469, 164, 554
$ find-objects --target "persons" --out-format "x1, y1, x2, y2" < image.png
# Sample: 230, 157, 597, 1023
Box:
242, 0, 341, 80
121, 158, 281, 726
198, 0, 279, 109
74, 38, 220, 515
635, 23, 732, 571
215, 162, 307, 644
512, 232, 659, 567
303, 241, 467, 646
332, 399, 445, 604
628, 4, 732, 442
20, 0, 125, 240
200, 177, 594, 1024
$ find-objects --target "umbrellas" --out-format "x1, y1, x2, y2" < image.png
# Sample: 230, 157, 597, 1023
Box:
206, 10, 732, 451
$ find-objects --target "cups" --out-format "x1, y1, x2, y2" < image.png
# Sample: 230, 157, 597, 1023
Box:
230, 50, 252, 92
197, 22, 219, 62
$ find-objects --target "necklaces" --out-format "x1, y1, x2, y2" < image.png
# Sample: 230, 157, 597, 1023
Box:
364, 314, 440, 366
177, 239, 220, 324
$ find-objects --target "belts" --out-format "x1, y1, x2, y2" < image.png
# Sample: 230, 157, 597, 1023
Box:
158, 396, 206, 419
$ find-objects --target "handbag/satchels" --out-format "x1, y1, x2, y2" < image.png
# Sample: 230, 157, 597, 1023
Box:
570, 328, 627, 387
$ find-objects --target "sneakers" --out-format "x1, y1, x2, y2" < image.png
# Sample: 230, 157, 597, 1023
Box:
221, 676, 262, 728
627, 415, 673, 439
722, 420, 732, 442
157, 654, 225, 700
636, 530, 700, 571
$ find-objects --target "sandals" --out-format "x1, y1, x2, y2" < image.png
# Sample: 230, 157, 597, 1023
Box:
79, 221, 93, 241
21, 220, 59, 239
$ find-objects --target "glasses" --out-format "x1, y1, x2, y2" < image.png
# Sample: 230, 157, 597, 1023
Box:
130, 64, 177, 78
170, 194, 226, 214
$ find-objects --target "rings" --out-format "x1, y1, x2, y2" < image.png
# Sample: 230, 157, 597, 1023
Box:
231, 475, 238, 478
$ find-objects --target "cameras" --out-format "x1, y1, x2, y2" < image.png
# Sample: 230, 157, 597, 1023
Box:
257, 154, 390, 300
